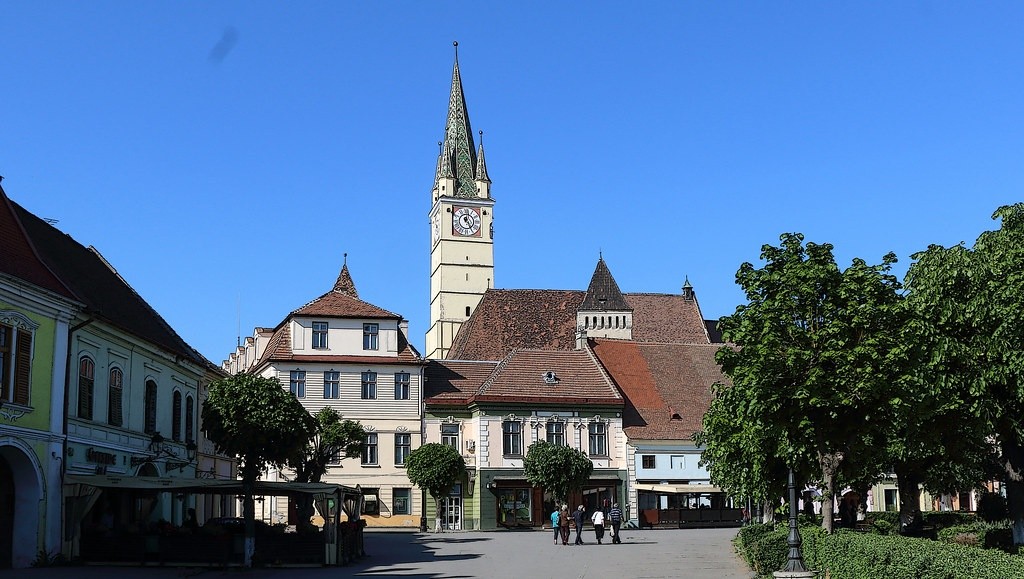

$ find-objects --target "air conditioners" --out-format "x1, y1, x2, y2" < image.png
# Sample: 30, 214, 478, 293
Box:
466, 439, 473, 450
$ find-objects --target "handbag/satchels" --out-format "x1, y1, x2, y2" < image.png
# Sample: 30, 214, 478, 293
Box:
609, 524, 615, 537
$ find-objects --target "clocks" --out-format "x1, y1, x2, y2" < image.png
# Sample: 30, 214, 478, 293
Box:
452, 205, 482, 237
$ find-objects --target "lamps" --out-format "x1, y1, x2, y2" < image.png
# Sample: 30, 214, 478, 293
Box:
149, 431, 164, 457
486, 481, 492, 489
185, 439, 197, 463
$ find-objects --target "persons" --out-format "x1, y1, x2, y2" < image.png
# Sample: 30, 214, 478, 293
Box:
572, 505, 585, 545
609, 503, 624, 543
184, 508, 197, 529
551, 506, 566, 545
559, 504, 572, 545
591, 505, 605, 544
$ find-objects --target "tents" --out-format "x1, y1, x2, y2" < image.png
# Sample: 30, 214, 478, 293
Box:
62, 474, 363, 568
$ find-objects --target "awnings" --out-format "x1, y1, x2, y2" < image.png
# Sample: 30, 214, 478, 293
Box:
634, 483, 737, 493
